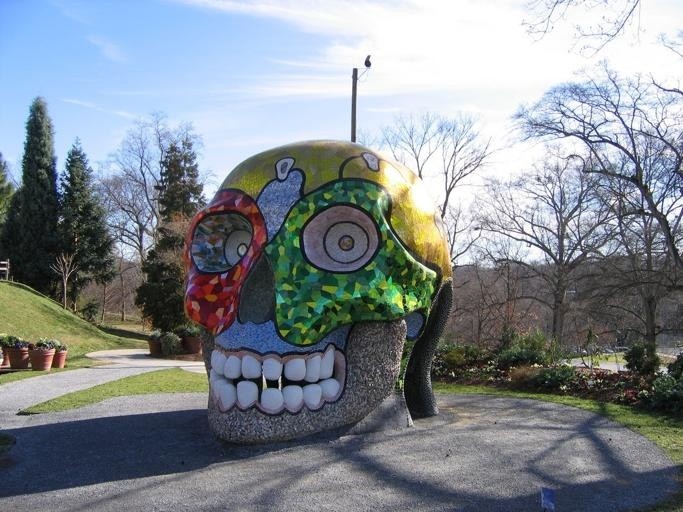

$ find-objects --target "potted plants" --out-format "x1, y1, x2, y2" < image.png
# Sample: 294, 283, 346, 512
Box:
145, 324, 202, 358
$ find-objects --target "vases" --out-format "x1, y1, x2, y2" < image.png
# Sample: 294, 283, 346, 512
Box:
0, 347, 66, 371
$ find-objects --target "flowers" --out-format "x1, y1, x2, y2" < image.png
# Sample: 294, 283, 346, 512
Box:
0, 333, 67, 353
